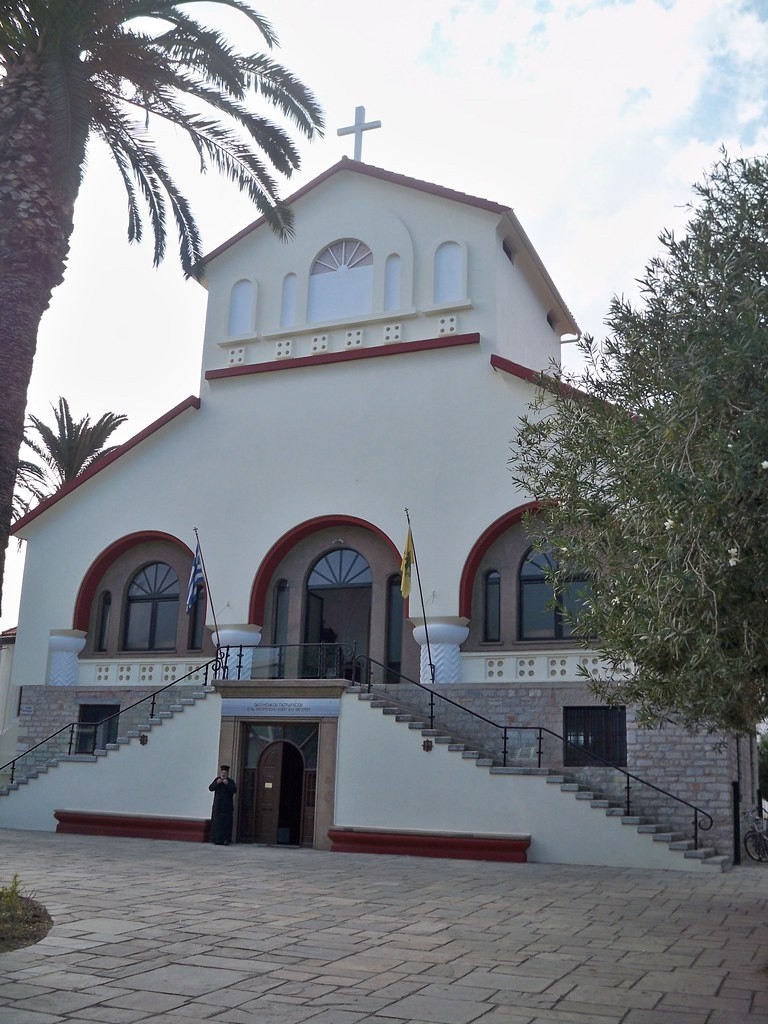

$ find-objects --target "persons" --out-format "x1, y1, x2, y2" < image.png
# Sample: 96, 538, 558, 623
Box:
209, 765, 237, 845
320, 638, 337, 678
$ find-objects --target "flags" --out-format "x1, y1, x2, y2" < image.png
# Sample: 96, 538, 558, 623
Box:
400, 526, 415, 599
185, 543, 205, 615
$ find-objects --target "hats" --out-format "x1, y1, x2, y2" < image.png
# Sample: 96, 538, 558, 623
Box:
221, 766, 230, 771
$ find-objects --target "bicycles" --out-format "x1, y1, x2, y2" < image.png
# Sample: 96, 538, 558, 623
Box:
742, 810, 768, 862
